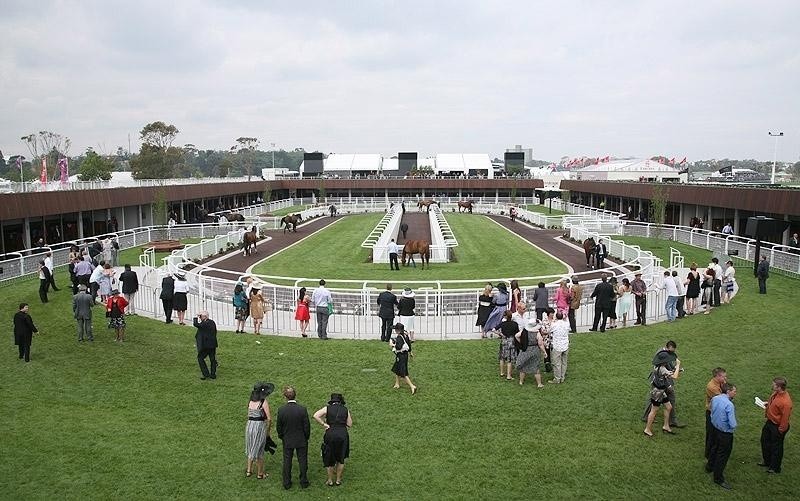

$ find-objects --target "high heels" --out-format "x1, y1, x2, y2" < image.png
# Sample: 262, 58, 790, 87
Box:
662, 426, 676, 435
643, 431, 654, 438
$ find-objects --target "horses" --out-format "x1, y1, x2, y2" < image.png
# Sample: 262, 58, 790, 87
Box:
458, 200, 474, 214
220, 212, 245, 230
583, 237, 596, 268
401, 239, 430, 271
280, 214, 302, 235
417, 200, 437, 212
240, 226, 257, 256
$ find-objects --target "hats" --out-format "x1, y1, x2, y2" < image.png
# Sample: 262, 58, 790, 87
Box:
572, 276, 578, 281
635, 272, 642, 275
250, 382, 274, 402
401, 289, 416, 298
608, 277, 618, 283
252, 281, 264, 290
524, 318, 542, 333
652, 350, 678, 366
111, 290, 120, 295
173, 272, 187, 281
79, 286, 88, 291
235, 282, 243, 287
389, 323, 404, 331
493, 283, 510, 295
328, 394, 346, 405
125, 265, 131, 272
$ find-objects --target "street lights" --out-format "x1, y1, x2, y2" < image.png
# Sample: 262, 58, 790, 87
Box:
767, 129, 788, 188
270, 140, 276, 165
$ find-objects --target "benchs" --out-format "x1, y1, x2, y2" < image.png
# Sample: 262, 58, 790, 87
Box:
140, 240, 185, 252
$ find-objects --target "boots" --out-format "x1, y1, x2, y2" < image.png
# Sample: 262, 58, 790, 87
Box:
589, 320, 647, 333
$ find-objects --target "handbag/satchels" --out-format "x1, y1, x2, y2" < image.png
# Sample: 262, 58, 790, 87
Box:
649, 387, 667, 405
520, 329, 528, 353
394, 343, 410, 355
106, 312, 112, 318
701, 281, 713, 289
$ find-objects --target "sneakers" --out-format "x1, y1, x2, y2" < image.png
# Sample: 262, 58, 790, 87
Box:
393, 384, 400, 390
569, 330, 575, 333
704, 461, 781, 490
641, 417, 659, 424
245, 468, 342, 490
200, 375, 216, 380
500, 364, 564, 389
704, 311, 710, 314
669, 420, 687, 428
664, 310, 694, 323
411, 386, 417, 395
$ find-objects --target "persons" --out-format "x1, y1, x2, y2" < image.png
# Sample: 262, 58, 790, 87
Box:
328, 200, 409, 271
232, 277, 417, 394
168, 202, 244, 225
313, 392, 352, 486
599, 203, 734, 234
701, 367, 728, 458
788, 234, 799, 253
13, 214, 218, 380
474, 239, 734, 389
276, 386, 311, 490
642, 350, 680, 438
757, 255, 770, 294
708, 384, 739, 490
756, 377, 792, 473
642, 340, 686, 428
469, 203, 516, 222
245, 380, 274, 479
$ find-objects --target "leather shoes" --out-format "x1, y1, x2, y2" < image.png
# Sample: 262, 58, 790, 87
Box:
236, 330, 260, 335
166, 319, 186, 326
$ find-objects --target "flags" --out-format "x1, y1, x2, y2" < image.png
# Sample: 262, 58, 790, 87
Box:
550, 156, 610, 171
58, 158, 68, 190
40, 157, 47, 190
16, 157, 21, 171
658, 156, 687, 166
